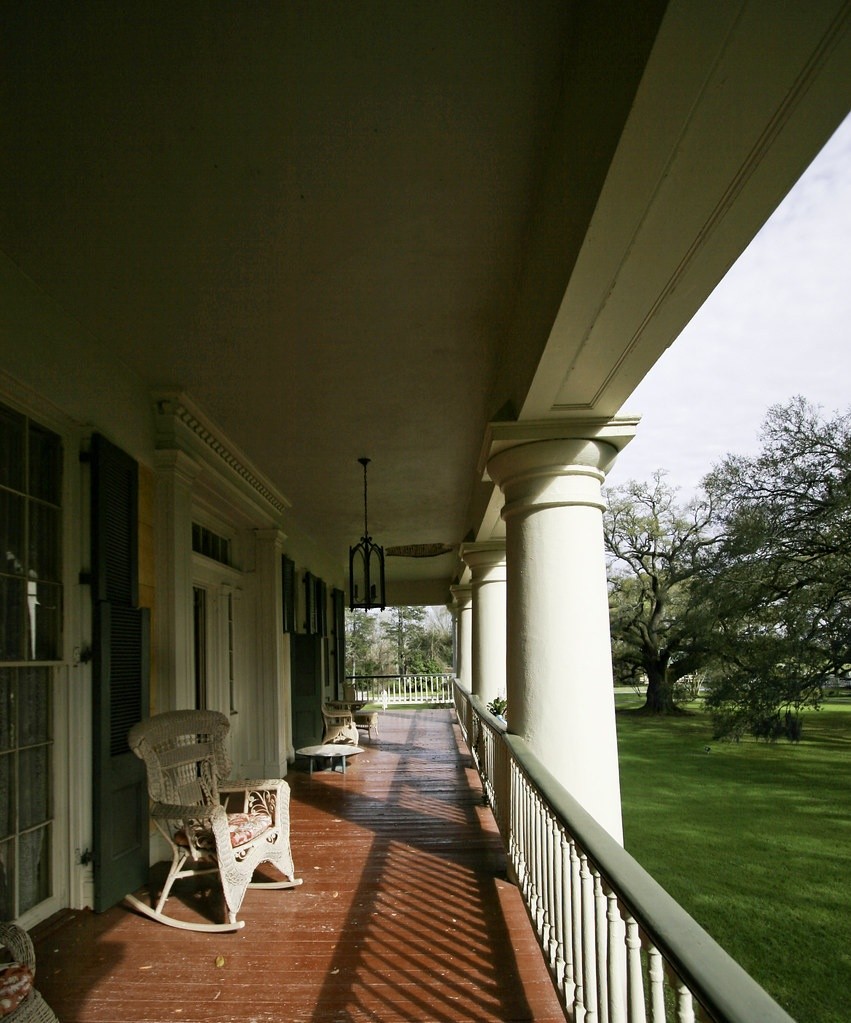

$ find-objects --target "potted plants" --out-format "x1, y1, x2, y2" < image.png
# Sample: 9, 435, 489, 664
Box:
487, 697, 508, 718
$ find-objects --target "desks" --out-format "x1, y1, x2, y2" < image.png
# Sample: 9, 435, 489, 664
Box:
353, 711, 378, 738
296, 745, 365, 774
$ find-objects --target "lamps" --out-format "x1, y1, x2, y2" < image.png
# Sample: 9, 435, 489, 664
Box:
350, 457, 387, 612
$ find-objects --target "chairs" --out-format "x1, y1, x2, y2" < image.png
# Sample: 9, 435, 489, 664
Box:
124, 710, 302, 932
344, 685, 367, 731
321, 702, 359, 745
0, 922, 60, 1023
